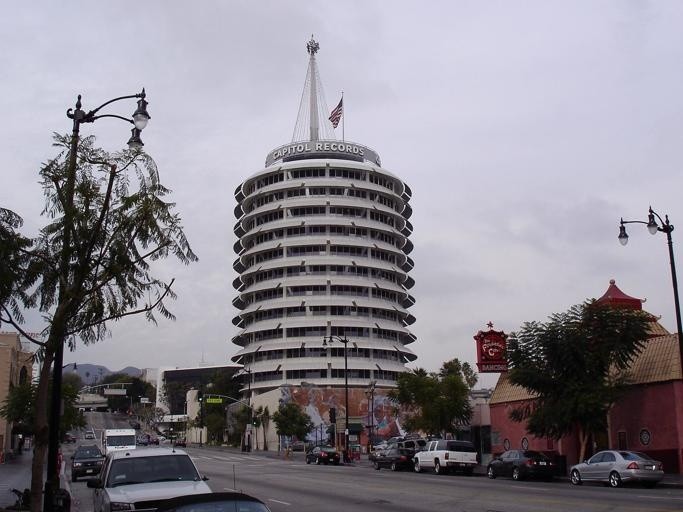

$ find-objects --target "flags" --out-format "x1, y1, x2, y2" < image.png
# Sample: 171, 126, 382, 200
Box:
328, 91, 344, 131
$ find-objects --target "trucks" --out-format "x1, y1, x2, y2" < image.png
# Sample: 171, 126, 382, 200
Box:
100, 428, 136, 456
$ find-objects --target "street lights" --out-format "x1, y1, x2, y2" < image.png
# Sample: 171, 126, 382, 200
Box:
369, 379, 376, 451
619, 206, 682, 370
322, 334, 348, 455
365, 389, 371, 454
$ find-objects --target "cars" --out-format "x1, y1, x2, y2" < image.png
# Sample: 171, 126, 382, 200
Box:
85, 431, 94, 439
137, 433, 165, 444
413, 440, 478, 473
372, 435, 428, 456
86, 446, 213, 512
375, 449, 412, 472
487, 450, 556, 481
306, 446, 340, 465
570, 449, 664, 488
71, 444, 104, 482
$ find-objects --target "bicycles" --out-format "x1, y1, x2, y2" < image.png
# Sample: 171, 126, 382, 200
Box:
12, 489, 25, 512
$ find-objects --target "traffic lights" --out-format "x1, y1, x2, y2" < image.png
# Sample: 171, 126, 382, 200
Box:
198, 391, 203, 401
328, 407, 335, 422
